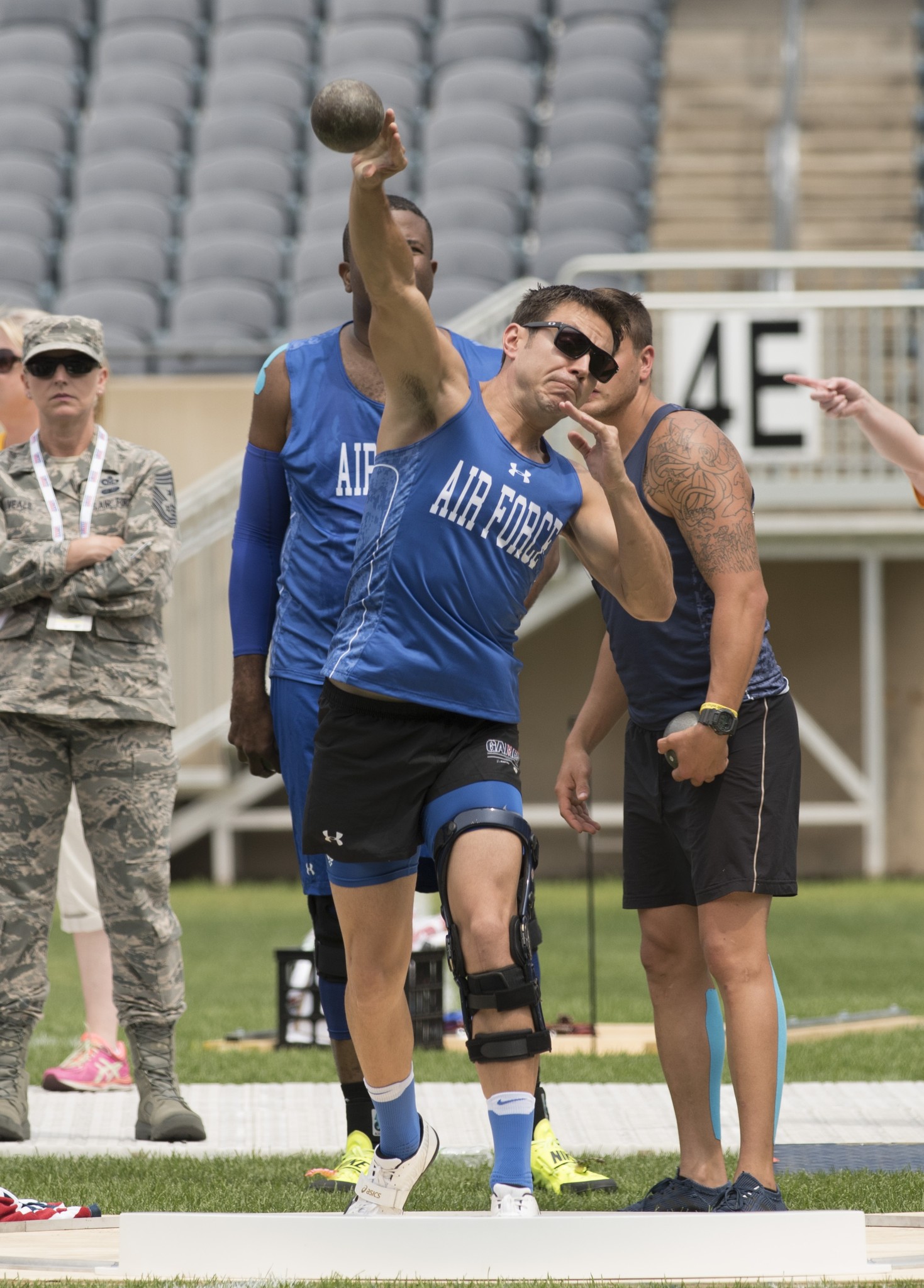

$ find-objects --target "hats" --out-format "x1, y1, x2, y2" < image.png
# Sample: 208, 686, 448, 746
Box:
22, 315, 104, 367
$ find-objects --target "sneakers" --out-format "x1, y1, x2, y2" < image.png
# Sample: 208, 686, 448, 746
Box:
344, 1113, 439, 1216
621, 1167, 730, 1212
491, 1183, 539, 1216
42, 1032, 136, 1092
709, 1171, 788, 1212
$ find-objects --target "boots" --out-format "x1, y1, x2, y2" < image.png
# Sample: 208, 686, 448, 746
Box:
0, 1014, 32, 1140
124, 1024, 206, 1140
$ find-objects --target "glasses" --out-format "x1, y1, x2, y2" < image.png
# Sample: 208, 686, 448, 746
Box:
24, 353, 99, 375
524, 319, 620, 383
0, 348, 22, 373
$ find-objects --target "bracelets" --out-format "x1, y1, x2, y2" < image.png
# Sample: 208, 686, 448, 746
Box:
698, 702, 739, 719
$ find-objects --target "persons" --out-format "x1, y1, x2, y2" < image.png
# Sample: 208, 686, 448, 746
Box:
783, 373, 924, 509
227, 195, 622, 1199
555, 286, 805, 1212
1, 309, 208, 1143
297, 107, 681, 1214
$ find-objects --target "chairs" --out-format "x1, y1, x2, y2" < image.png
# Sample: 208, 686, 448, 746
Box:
1, 1, 667, 374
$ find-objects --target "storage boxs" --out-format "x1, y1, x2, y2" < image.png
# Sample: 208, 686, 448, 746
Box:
274, 948, 446, 1052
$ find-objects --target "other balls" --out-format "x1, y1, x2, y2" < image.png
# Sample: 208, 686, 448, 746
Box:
309, 77, 385, 154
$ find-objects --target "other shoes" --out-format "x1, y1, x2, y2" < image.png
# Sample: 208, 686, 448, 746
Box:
322, 1130, 376, 1191
531, 1118, 616, 1193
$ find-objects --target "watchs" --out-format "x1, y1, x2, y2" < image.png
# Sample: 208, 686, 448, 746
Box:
697, 707, 739, 736
